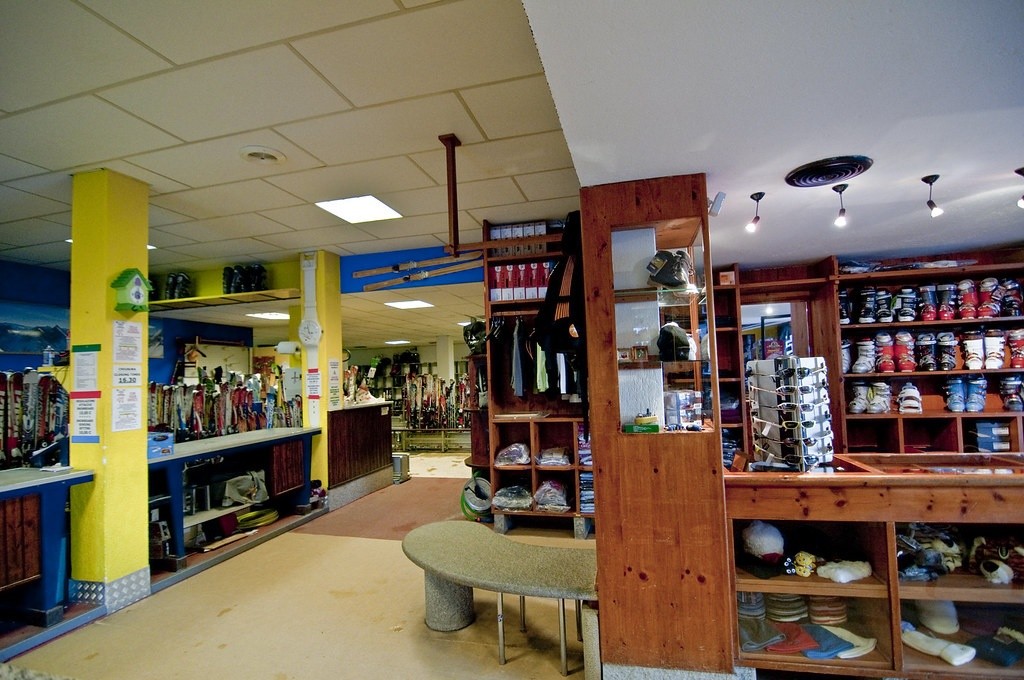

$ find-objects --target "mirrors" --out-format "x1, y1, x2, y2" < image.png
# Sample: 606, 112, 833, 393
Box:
741, 300, 813, 454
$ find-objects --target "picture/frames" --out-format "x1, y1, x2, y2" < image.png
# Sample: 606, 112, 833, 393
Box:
615, 345, 649, 362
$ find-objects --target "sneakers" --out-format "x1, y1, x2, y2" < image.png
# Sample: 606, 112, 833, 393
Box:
847, 374, 1024, 415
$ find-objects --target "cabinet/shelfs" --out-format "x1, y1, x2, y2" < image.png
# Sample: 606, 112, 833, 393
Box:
580, 171, 734, 674
482, 218, 595, 540
462, 354, 493, 523
726, 451, 1024, 680
148, 427, 322, 573
349, 362, 470, 452
664, 254, 1024, 466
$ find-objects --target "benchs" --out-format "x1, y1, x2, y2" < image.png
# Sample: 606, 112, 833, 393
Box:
402, 520, 600, 677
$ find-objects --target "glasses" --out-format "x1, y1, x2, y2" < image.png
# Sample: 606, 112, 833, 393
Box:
748, 364, 834, 473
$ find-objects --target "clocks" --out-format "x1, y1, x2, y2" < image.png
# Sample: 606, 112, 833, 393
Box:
298, 319, 321, 346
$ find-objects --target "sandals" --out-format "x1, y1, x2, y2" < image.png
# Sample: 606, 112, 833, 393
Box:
875, 332, 895, 372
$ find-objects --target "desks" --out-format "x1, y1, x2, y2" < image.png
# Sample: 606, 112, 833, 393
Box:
0, 467, 95, 629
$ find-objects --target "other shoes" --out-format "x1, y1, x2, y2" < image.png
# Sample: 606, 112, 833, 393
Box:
647, 249, 691, 287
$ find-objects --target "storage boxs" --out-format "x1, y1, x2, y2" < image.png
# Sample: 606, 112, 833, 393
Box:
664, 388, 702, 429
625, 422, 660, 433
719, 270, 734, 286
489, 220, 547, 257
149, 508, 172, 559
967, 422, 1012, 453
488, 259, 551, 301
147, 431, 175, 460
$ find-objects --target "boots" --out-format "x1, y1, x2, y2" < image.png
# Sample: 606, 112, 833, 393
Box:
840, 339, 851, 373
960, 330, 984, 370
937, 284, 957, 320
858, 286, 876, 324
983, 329, 1006, 369
1001, 277, 1023, 317
914, 333, 937, 371
1008, 329, 1023, 368
978, 278, 1001, 318
837, 290, 852, 324
957, 279, 979, 318
851, 338, 877, 373
894, 332, 918, 371
920, 284, 937, 321
936, 331, 958, 371
876, 287, 894, 321
895, 286, 918, 321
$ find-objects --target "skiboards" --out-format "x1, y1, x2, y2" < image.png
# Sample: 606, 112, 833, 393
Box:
405, 371, 471, 436
148, 364, 275, 442
0, 367, 69, 470
276, 364, 302, 428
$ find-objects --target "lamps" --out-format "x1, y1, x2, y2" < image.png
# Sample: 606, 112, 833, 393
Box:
1015, 166, 1024, 208
745, 191, 765, 233
922, 174, 943, 217
833, 183, 848, 228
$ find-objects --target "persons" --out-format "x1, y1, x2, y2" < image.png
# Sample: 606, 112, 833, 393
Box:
355, 375, 377, 404
342, 382, 349, 398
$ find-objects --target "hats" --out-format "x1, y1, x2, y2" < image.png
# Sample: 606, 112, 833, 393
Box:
737, 594, 878, 660
657, 324, 694, 361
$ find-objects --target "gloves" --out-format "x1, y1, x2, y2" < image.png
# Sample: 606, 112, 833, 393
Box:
901, 628, 976, 665
